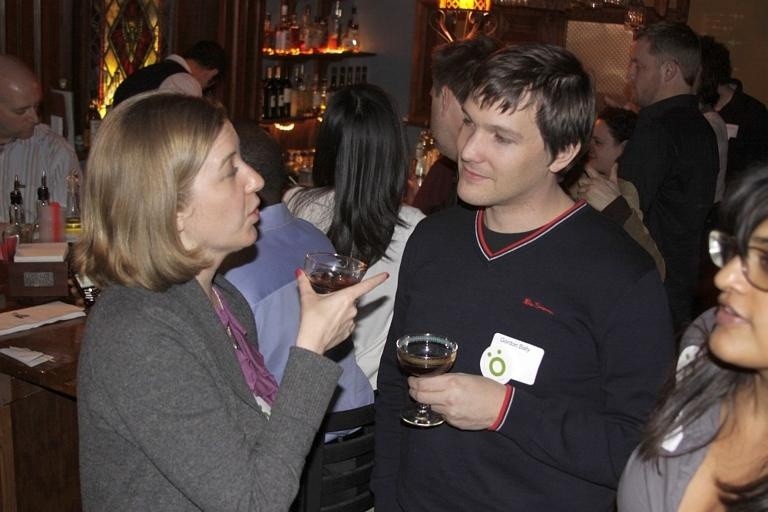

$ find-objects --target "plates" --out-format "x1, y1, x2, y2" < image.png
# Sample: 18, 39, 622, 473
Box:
257, 52, 378, 124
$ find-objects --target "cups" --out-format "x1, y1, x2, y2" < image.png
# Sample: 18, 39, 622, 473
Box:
708, 230, 768, 294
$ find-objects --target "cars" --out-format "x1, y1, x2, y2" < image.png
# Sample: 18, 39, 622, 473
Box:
37, 203, 65, 240
299, 250, 364, 295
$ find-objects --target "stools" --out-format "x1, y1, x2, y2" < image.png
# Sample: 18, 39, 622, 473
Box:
325, 427, 364, 445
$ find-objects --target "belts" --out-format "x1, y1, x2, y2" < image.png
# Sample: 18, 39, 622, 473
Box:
392, 331, 459, 434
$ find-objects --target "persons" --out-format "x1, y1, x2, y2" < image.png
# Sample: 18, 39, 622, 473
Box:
70, 90, 390, 511
369, 44, 676, 510
2, 16, 767, 444
616, 164, 767, 510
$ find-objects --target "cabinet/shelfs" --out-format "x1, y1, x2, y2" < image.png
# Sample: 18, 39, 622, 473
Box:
263, 2, 366, 58
8, 173, 81, 245
403, 133, 439, 200
260, 61, 371, 118
280, 147, 316, 176
